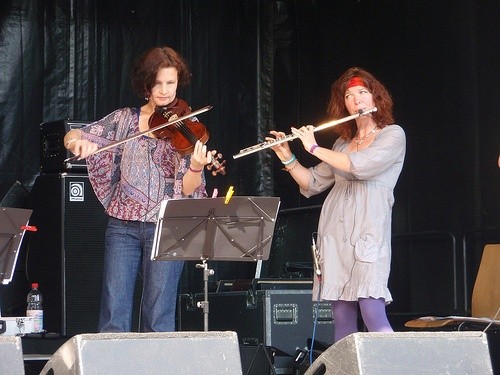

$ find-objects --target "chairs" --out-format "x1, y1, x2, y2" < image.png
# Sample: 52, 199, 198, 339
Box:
405, 244, 500, 331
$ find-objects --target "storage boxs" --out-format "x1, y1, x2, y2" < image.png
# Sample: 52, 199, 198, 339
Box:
178, 290, 335, 374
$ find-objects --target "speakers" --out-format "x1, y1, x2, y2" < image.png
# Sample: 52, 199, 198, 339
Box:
0, 336, 25, 375
304, 330, 494, 375
39, 330, 243, 375
30, 171, 145, 335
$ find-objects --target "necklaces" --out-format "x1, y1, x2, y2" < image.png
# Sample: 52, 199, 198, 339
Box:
356, 127, 376, 145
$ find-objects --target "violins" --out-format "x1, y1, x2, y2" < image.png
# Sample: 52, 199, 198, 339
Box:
149, 98, 227, 176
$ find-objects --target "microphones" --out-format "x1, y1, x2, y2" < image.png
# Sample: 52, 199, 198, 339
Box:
312, 236, 321, 278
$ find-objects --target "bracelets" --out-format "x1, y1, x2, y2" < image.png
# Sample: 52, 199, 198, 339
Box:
285, 160, 298, 171
309, 144, 318, 154
66, 138, 76, 150
282, 154, 296, 165
190, 167, 202, 172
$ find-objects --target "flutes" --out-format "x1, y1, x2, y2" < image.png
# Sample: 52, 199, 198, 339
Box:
233, 107, 378, 159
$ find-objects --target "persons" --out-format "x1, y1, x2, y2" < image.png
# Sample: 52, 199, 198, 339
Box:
63, 47, 212, 333
263, 68, 405, 346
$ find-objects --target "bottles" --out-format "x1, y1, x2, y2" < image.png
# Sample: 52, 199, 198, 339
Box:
27, 283, 44, 334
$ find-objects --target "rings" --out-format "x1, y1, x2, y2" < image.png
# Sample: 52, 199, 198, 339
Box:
300, 132, 303, 135
